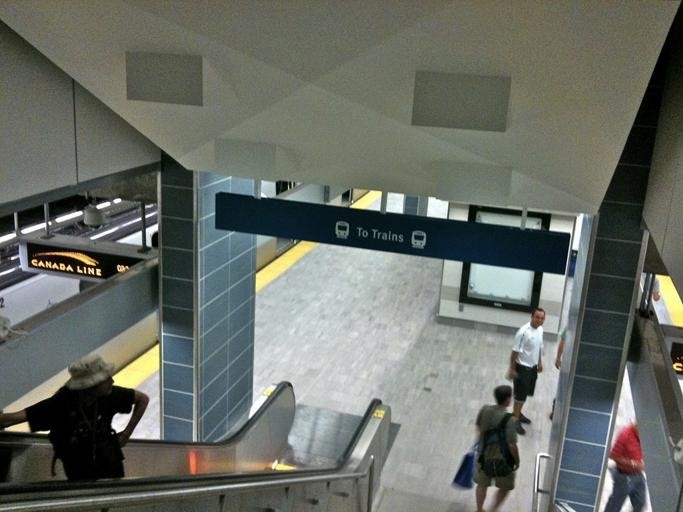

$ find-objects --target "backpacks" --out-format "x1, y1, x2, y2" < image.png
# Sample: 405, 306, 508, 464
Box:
480, 413, 515, 477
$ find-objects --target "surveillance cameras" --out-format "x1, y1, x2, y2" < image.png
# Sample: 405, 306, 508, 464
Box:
83, 207, 107, 230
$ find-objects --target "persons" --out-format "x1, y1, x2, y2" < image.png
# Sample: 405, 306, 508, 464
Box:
640, 271, 662, 301
475, 385, 521, 512
505, 308, 544, 436
0, 353, 149, 481
603, 424, 647, 512
549, 325, 569, 420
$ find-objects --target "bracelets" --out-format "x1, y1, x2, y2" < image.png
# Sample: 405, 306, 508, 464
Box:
631, 459, 637, 468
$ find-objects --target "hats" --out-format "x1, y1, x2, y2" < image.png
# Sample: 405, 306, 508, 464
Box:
65, 354, 114, 390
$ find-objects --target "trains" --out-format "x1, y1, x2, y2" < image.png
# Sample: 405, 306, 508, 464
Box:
0, 181, 370, 415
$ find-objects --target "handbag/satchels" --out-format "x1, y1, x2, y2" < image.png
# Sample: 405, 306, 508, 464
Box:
453, 452, 473, 488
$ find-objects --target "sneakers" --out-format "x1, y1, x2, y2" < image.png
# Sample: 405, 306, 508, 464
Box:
513, 411, 530, 434
